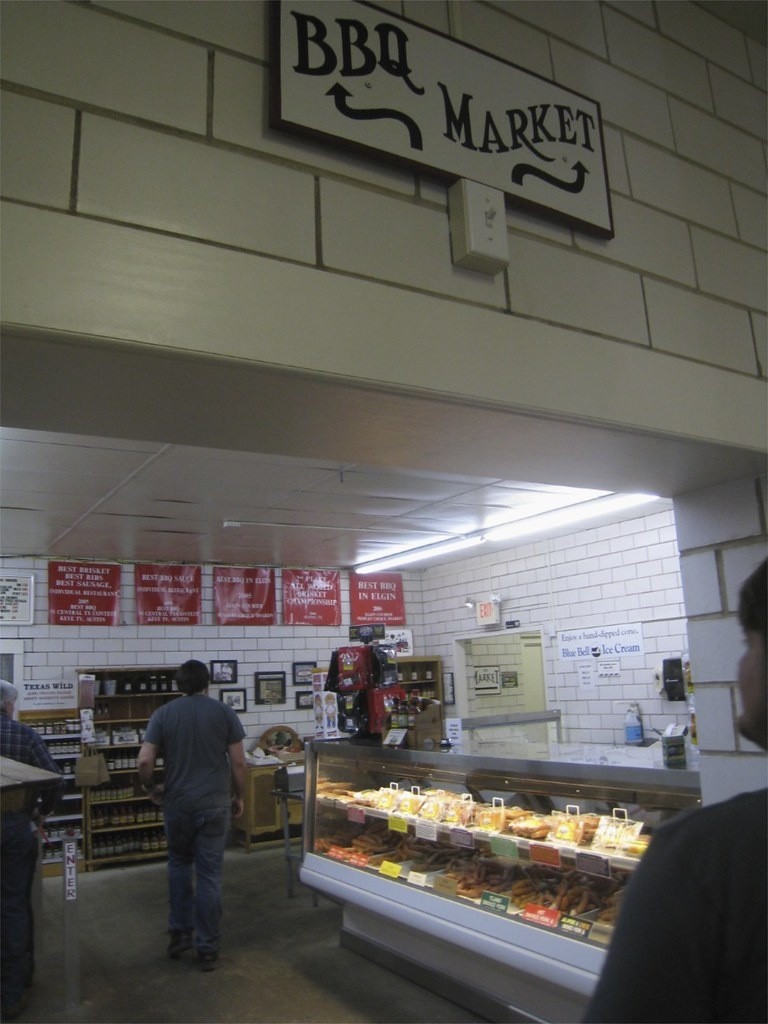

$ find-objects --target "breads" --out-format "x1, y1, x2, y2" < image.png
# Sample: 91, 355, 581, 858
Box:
335, 786, 650, 856
317, 776, 354, 793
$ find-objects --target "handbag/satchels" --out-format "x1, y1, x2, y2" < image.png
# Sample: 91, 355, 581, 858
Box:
75, 746, 99, 786
86, 746, 111, 783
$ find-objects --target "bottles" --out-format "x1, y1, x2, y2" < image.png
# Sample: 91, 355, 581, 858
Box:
26, 719, 81, 773
41, 824, 82, 858
397, 666, 403, 682
91, 752, 168, 858
624, 709, 643, 744
411, 664, 417, 681
426, 663, 432, 679
390, 683, 436, 730
124, 675, 167, 692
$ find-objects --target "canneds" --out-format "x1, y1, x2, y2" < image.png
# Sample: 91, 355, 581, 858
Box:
121, 675, 179, 694
27, 718, 82, 861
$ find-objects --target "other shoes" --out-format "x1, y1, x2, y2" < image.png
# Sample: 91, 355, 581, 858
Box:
167, 934, 193, 955
196, 946, 218, 968
0, 989, 29, 1020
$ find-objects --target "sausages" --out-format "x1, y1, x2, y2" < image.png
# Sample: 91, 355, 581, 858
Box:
327, 824, 646, 927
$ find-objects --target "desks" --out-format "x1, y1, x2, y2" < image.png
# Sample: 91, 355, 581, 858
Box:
268, 788, 319, 908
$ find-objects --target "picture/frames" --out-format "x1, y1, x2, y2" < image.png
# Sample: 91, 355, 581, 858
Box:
254, 671, 286, 704
293, 661, 317, 685
220, 688, 247, 712
210, 660, 238, 683
296, 691, 314, 709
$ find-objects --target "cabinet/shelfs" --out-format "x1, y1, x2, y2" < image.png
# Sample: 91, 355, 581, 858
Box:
74, 666, 186, 872
380, 655, 445, 745
227, 750, 306, 852
20, 709, 87, 877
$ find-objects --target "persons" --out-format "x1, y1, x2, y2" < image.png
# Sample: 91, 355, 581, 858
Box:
139, 660, 247, 969
0, 681, 64, 1023
582, 557, 768, 1024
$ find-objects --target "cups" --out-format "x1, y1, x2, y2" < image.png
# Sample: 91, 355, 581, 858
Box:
104, 679, 117, 695
94, 680, 101, 695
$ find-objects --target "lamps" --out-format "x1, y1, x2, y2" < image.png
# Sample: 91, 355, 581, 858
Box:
484, 492, 659, 544
352, 533, 485, 575
